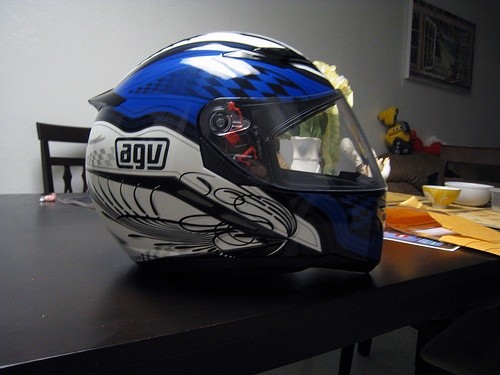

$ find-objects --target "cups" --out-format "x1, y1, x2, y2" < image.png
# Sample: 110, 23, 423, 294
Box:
490, 188, 500, 211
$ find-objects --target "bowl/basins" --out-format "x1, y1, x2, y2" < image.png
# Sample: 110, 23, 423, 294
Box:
421, 185, 462, 209
445, 182, 495, 207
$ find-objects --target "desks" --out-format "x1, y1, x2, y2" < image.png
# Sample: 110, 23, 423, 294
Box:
0, 185, 500, 374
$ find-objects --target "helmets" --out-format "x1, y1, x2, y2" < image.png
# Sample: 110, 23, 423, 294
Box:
86, 32, 387, 272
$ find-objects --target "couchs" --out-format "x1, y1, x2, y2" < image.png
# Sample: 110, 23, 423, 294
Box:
355, 144, 500, 197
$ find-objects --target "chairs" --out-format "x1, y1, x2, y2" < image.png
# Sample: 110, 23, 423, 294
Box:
35, 122, 92, 197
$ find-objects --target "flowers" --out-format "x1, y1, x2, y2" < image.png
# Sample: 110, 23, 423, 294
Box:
284, 59, 354, 137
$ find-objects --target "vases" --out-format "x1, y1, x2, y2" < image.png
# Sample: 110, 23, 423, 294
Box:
290, 135, 322, 174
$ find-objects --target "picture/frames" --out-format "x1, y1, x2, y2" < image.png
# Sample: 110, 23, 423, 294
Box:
403, 0, 479, 94
